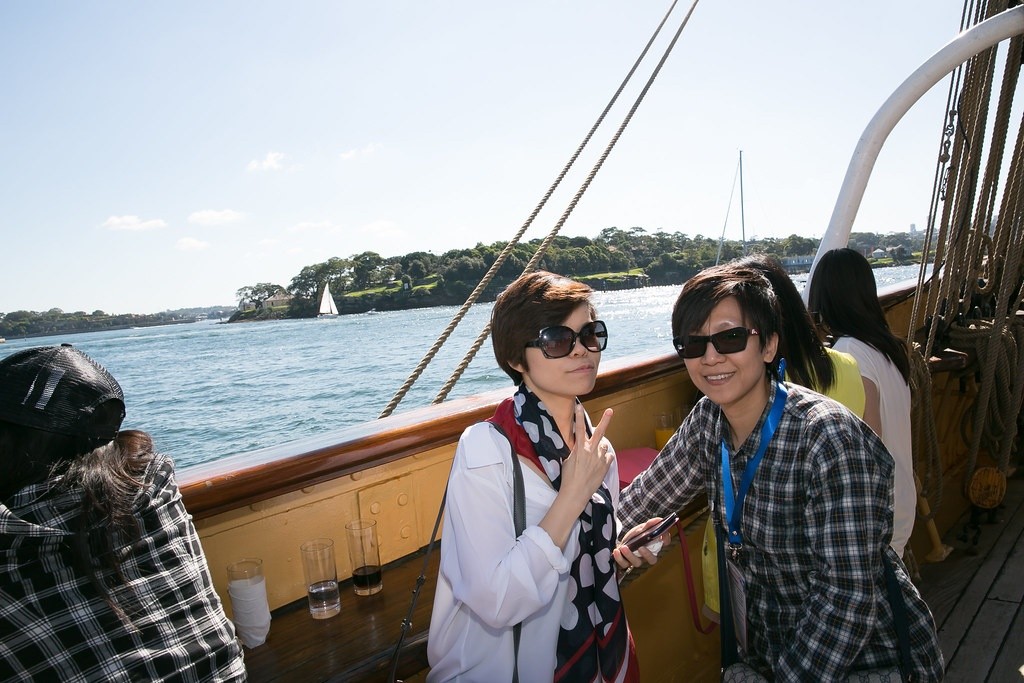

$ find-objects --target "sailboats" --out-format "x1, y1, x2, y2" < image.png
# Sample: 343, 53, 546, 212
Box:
716, 150, 807, 296
316, 282, 339, 319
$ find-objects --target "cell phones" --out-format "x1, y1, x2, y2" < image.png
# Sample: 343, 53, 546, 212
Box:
610, 513, 678, 560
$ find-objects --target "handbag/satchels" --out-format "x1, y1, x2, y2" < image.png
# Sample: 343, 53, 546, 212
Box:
721, 660, 903, 683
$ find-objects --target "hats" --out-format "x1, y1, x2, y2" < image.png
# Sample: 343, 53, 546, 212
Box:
1, 343, 128, 452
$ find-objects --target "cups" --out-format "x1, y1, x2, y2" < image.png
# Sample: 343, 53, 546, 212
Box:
301, 538, 341, 619
675, 407, 690, 427
655, 414, 674, 450
226, 558, 262, 584
345, 518, 383, 595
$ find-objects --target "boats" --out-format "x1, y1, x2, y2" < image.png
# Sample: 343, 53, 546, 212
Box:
367, 308, 379, 315
0, 335, 6, 343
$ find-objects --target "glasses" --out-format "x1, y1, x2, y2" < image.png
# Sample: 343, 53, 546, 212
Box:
673, 326, 760, 361
524, 320, 611, 361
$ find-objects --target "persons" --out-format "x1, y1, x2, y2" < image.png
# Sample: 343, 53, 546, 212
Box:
806, 249, 918, 559
0, 342, 247, 683
617, 265, 946, 683
701, 256, 867, 616
424, 270, 671, 683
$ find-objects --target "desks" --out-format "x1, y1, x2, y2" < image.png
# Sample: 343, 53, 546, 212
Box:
242, 548, 442, 683
